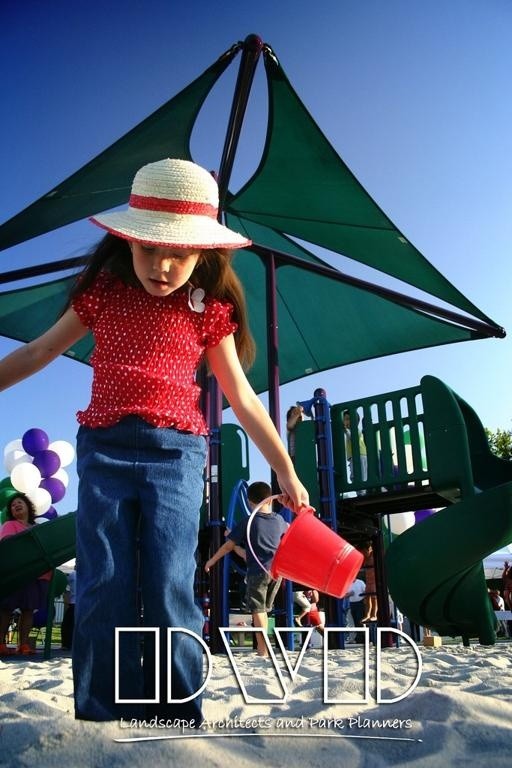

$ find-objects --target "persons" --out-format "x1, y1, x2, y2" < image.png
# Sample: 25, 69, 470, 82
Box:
286, 404, 305, 465
359, 541, 378, 624
289, 577, 433, 645
0, 155, 314, 727
0, 494, 40, 658
343, 409, 368, 497
62, 561, 76, 649
486, 561, 512, 641
224, 526, 248, 562
203, 481, 290, 656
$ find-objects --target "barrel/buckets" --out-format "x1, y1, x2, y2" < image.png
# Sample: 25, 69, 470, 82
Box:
245, 493, 365, 600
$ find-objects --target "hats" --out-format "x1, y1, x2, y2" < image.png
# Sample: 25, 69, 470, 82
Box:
84, 154, 257, 255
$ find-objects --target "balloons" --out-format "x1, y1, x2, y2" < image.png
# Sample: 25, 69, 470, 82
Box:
1, 427, 78, 627
358, 421, 444, 534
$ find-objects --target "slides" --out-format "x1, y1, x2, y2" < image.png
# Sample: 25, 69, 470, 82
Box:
385, 375, 512, 645
0, 512, 76, 605
